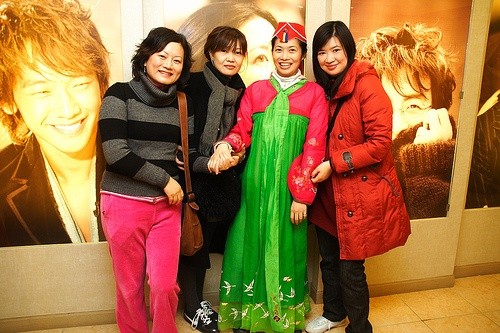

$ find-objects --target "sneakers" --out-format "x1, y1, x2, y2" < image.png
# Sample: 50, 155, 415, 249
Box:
183, 299, 219, 333
305, 316, 348, 333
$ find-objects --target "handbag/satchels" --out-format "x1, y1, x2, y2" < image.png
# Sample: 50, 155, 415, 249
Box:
180, 203, 203, 257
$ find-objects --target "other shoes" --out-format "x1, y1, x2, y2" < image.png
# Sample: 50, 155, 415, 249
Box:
233, 328, 245, 333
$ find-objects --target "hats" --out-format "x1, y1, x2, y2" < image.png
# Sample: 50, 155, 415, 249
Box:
272, 22, 306, 43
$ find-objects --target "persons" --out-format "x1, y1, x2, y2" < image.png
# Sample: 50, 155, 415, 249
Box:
173, 0, 285, 87
352, 23, 457, 221
0, 0, 111, 248
207, 22, 327, 333
174, 27, 253, 333
311, 20, 411, 333
99, 26, 233, 333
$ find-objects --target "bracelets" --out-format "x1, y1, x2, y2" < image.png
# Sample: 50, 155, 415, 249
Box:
213, 141, 235, 152
